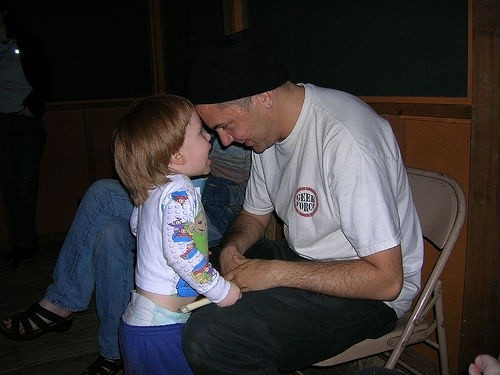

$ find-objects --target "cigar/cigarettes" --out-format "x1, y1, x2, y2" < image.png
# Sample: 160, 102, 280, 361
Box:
176, 296, 212, 315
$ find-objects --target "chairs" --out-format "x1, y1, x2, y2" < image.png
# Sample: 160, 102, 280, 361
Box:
313, 167, 467, 375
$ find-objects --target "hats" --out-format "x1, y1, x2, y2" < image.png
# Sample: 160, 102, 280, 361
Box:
183, 28, 290, 105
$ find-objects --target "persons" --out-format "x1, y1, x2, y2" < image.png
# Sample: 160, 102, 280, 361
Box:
468, 352, 500, 375
181, 30, 425, 375
111, 95, 244, 375
1, 131, 252, 375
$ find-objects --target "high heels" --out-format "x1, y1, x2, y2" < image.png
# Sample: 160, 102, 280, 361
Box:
0, 304, 75, 341
82, 355, 124, 375
